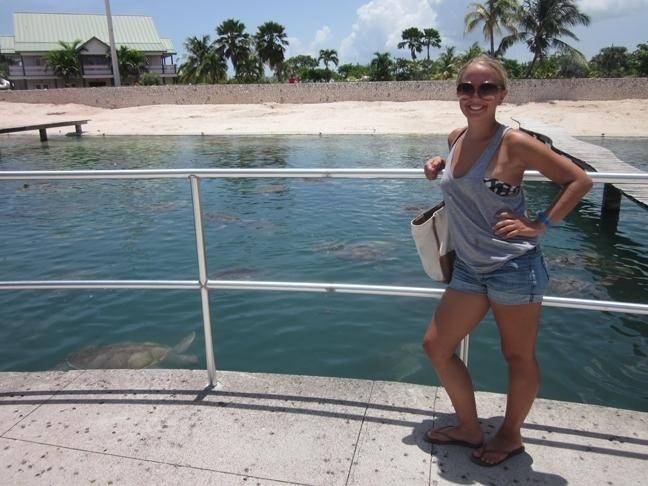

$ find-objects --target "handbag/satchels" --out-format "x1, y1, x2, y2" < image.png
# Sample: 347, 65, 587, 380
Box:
410, 199, 454, 283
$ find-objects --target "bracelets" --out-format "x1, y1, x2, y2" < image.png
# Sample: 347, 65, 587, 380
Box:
535, 211, 553, 231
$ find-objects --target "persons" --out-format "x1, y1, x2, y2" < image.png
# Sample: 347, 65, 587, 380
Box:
421, 53, 596, 470
288, 74, 297, 82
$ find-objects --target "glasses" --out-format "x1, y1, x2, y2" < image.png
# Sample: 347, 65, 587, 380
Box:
456, 82, 501, 101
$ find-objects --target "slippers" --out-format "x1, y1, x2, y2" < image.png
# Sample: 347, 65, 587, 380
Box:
423, 426, 484, 449
471, 439, 525, 467
